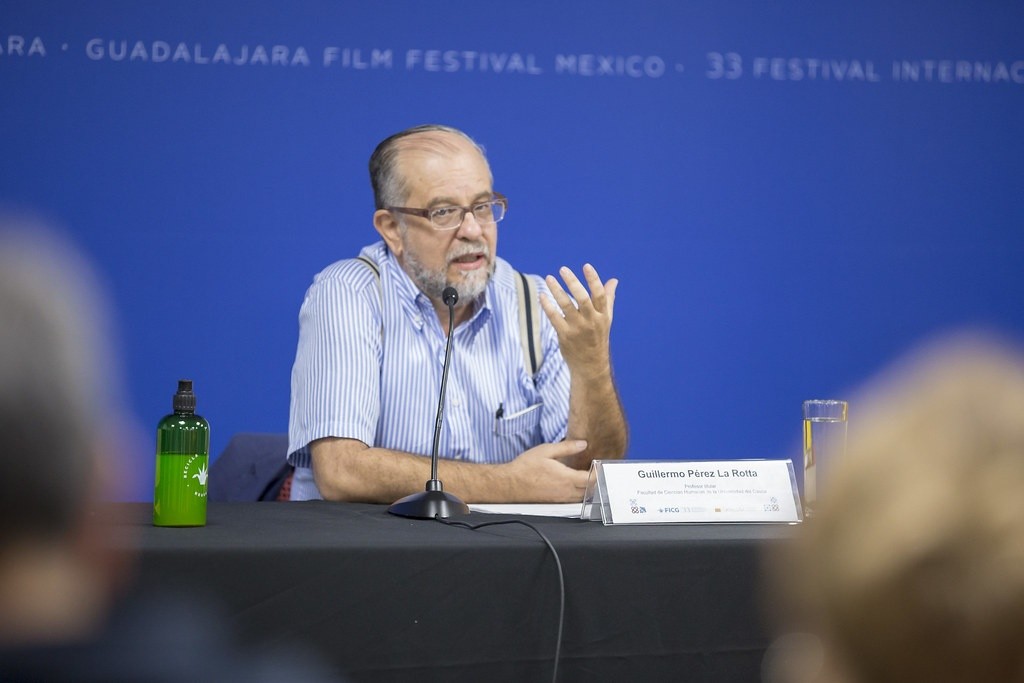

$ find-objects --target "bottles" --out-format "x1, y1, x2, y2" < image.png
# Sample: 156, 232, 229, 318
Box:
152, 380, 210, 527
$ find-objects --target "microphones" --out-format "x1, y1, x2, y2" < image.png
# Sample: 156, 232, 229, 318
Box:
390, 287, 470, 520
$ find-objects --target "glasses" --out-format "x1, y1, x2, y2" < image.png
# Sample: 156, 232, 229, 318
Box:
388, 191, 510, 230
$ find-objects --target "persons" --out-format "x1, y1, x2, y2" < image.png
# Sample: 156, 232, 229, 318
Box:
1, 215, 161, 683
765, 330, 1021, 683
287, 124, 627, 502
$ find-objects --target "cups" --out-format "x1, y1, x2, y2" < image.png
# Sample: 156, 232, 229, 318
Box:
802, 399, 849, 518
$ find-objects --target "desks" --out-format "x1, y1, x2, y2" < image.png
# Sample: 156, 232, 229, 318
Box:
87, 503, 803, 683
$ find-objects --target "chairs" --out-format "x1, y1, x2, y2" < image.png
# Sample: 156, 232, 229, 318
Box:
207, 435, 295, 502
